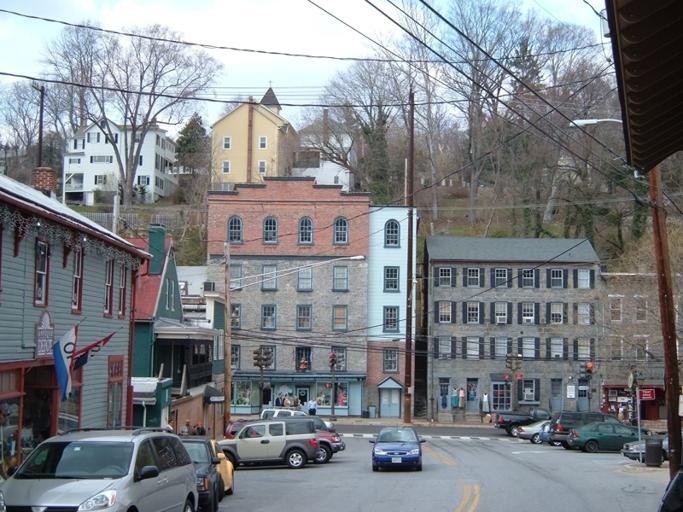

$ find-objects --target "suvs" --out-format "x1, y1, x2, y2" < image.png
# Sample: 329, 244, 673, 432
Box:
279, 416, 328, 431
548, 410, 622, 450
259, 408, 337, 432
225, 420, 345, 465
0, 425, 199, 512
216, 418, 320, 468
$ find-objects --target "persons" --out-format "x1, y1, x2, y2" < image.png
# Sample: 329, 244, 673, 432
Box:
481, 392, 489, 413
449, 385, 458, 410
166, 418, 206, 439
275, 389, 347, 415
457, 388, 464, 408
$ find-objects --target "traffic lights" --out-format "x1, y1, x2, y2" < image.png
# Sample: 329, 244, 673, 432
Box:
253, 347, 262, 368
514, 354, 522, 370
328, 352, 337, 369
505, 353, 513, 370
262, 347, 272, 367
583, 362, 593, 381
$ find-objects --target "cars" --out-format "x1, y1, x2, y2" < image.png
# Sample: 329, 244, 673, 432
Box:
179, 436, 225, 512
516, 419, 551, 445
207, 438, 234, 497
569, 421, 650, 454
368, 425, 426, 471
539, 422, 561, 447
619, 433, 669, 462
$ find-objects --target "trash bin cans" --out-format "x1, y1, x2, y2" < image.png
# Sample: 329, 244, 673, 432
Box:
646, 438, 662, 466
369, 406, 376, 418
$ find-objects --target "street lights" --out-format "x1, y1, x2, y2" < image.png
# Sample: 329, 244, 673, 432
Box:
566, 115, 681, 485
222, 239, 364, 427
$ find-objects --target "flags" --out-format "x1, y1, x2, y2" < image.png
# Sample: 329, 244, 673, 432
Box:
71, 332, 117, 371
53, 325, 77, 402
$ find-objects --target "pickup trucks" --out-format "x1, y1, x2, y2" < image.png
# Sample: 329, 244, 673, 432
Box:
491, 408, 550, 438
